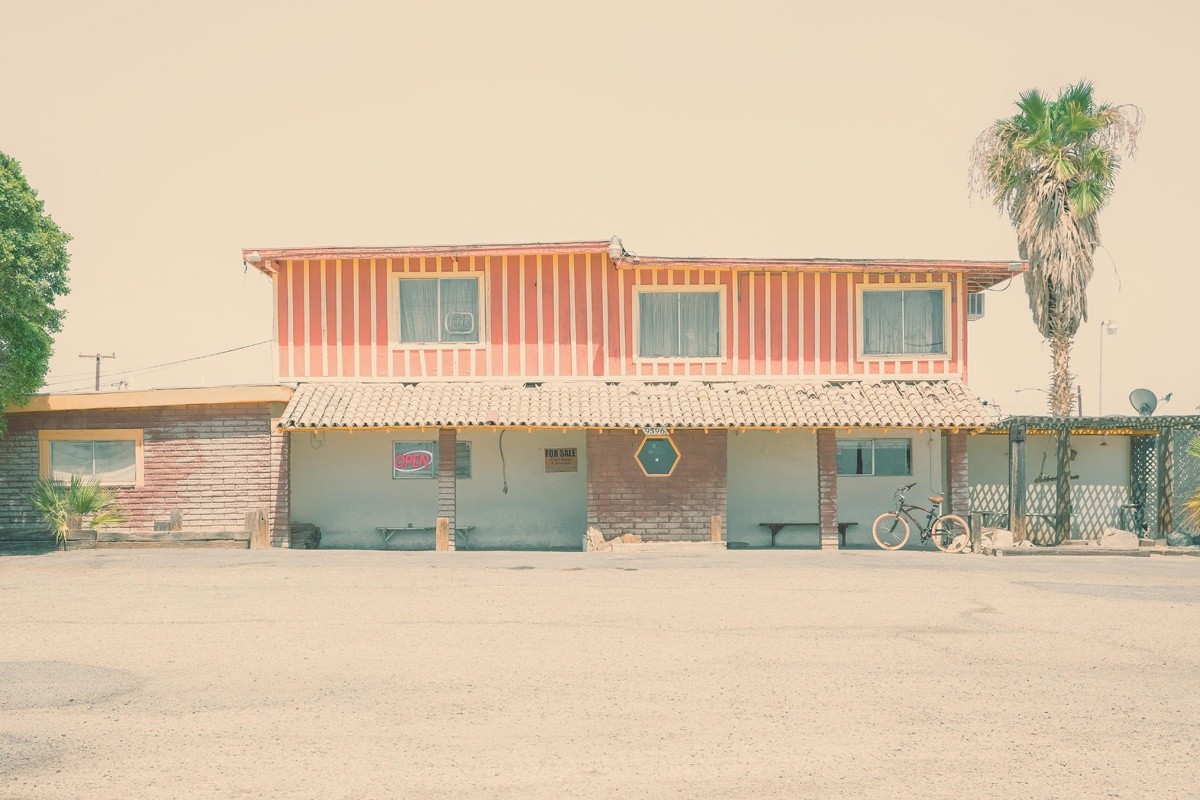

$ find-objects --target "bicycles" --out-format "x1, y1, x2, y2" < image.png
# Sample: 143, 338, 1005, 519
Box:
871, 481, 972, 553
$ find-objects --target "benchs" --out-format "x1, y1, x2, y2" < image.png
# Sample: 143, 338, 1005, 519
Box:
377, 525, 475, 551
972, 511, 1079, 548
759, 522, 858, 546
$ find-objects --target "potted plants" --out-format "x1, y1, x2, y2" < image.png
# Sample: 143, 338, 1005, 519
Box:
10, 475, 129, 549
